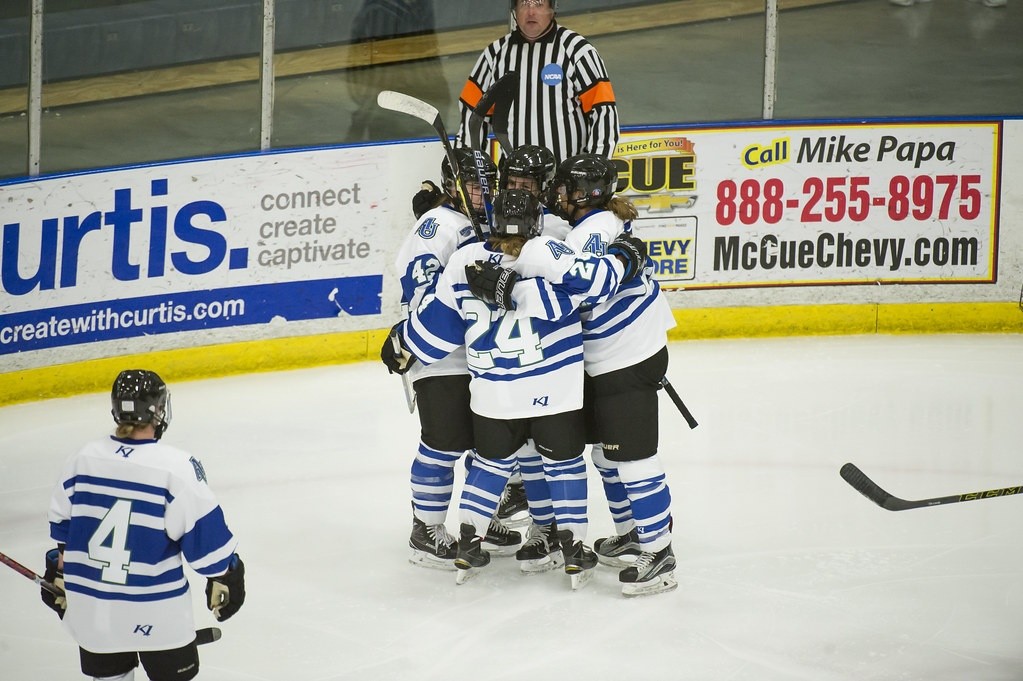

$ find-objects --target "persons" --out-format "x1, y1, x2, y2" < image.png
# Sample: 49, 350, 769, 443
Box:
41, 369, 246, 681
453, 0, 619, 169
382, 144, 677, 595
348, 0, 450, 139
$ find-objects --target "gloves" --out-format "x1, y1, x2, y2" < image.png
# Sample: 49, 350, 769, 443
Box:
41, 548, 68, 621
381, 319, 417, 376
204, 552, 246, 623
608, 233, 647, 284
464, 259, 522, 311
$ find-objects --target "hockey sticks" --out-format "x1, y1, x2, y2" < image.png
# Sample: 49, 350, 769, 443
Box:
0, 552, 222, 646
375, 89, 483, 241
468, 72, 520, 234
491, 71, 699, 430
839, 462, 1023, 512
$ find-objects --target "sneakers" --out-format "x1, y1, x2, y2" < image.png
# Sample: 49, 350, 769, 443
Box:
594, 522, 641, 568
619, 516, 679, 598
516, 519, 565, 577
454, 522, 491, 584
555, 529, 598, 589
407, 500, 458, 571
494, 473, 533, 529
481, 515, 522, 556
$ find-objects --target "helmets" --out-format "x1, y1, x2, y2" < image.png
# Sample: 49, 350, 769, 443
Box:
510, 0, 558, 14
111, 369, 172, 439
490, 189, 544, 239
441, 146, 498, 224
498, 145, 555, 203
548, 153, 619, 226
